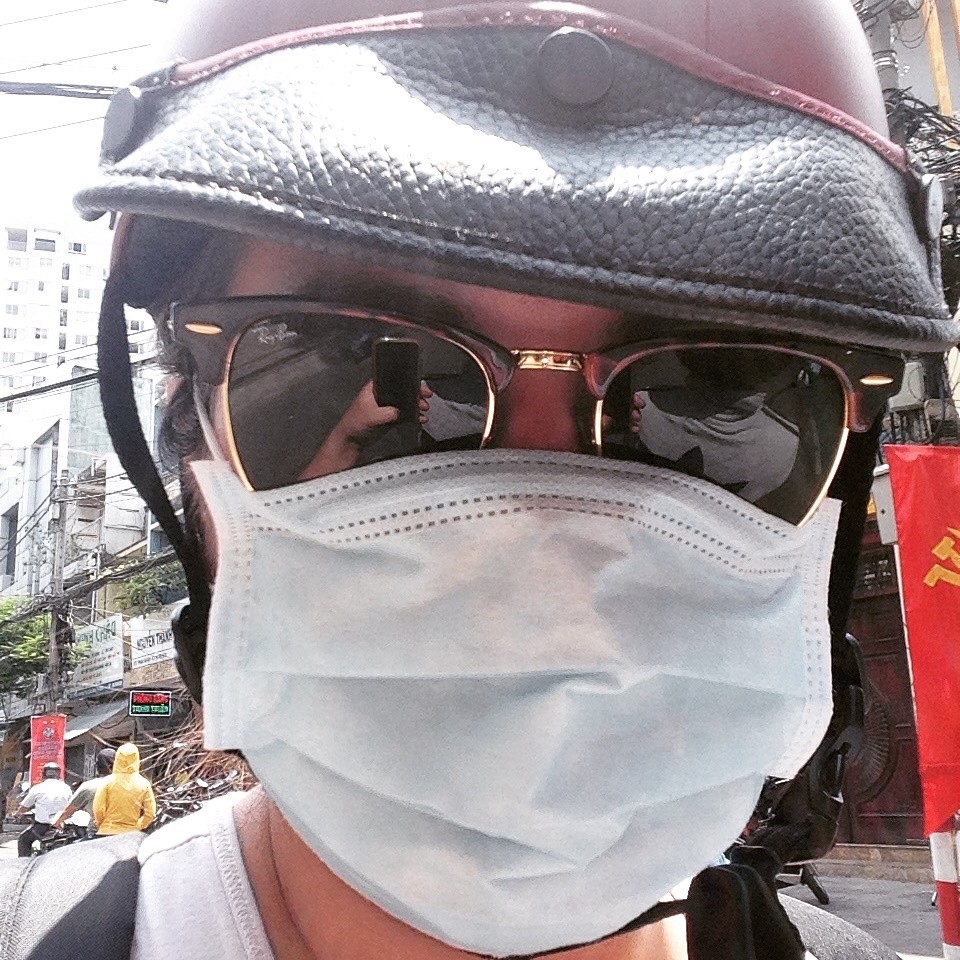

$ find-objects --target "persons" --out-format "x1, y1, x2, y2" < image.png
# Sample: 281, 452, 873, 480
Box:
0, 0, 959, 960
91, 742, 156, 839
49, 748, 116, 842
16, 761, 73, 857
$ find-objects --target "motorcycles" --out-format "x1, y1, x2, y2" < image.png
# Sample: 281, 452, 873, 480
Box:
13, 812, 97, 857
726, 837, 826, 902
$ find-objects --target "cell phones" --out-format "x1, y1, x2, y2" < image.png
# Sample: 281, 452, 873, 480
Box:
371, 337, 422, 426
602, 366, 633, 434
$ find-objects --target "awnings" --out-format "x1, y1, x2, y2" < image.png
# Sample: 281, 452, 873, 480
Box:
22, 700, 129, 742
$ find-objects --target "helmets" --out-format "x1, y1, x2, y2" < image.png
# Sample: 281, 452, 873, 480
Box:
42, 763, 62, 775
73, 0, 959, 355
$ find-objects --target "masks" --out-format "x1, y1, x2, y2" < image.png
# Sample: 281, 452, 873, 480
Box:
191, 458, 840, 958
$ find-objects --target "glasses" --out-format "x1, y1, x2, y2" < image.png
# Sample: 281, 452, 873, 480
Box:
154, 297, 902, 525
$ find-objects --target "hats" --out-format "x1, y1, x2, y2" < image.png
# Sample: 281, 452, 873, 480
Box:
96, 749, 116, 771
